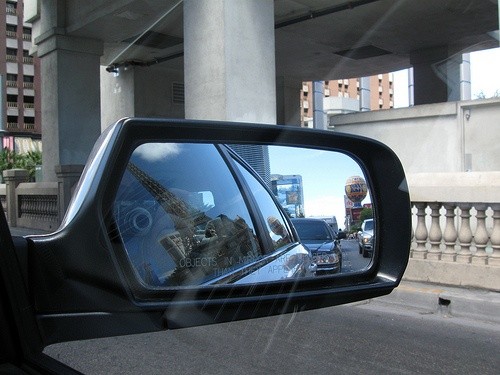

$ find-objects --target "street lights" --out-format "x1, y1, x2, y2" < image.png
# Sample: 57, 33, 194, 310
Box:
1, 129, 16, 169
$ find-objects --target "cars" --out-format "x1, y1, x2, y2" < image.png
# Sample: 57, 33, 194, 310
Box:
356, 219, 375, 258
289, 217, 344, 276
112, 139, 318, 287
311, 214, 362, 239
